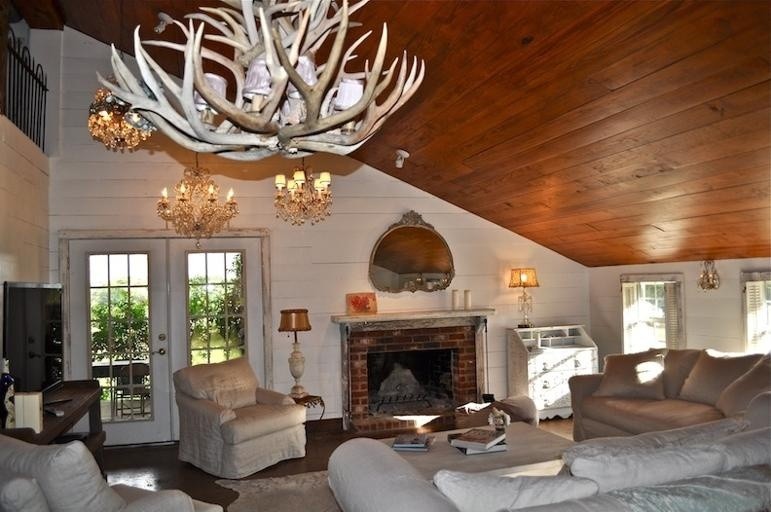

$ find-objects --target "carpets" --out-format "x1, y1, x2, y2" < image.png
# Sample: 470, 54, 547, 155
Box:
216, 469, 340, 512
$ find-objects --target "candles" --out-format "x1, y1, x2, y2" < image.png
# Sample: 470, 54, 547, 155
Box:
453, 289, 460, 309
464, 289, 475, 308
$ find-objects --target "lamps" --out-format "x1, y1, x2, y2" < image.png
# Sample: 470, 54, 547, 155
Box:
88, 47, 156, 153
156, 153, 241, 248
509, 268, 539, 328
696, 261, 721, 295
277, 310, 312, 396
273, 157, 333, 226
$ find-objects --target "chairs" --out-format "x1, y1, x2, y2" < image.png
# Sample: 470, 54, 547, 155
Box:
114, 363, 150, 419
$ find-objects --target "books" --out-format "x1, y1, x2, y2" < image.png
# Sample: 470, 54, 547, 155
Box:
390, 433, 436, 452
450, 429, 506, 451
447, 433, 507, 456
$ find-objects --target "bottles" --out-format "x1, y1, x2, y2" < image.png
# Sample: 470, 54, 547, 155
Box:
0, 358, 16, 429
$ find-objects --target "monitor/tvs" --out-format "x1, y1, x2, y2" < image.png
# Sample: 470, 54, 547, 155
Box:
2, 281, 73, 418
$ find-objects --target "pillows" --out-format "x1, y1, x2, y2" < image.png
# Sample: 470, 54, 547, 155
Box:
562, 418, 752, 493
0, 436, 126, 510
715, 351, 771, 416
600, 349, 669, 400
432, 462, 599, 512
678, 348, 764, 405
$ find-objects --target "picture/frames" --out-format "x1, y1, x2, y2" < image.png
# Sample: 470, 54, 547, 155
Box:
344, 293, 378, 315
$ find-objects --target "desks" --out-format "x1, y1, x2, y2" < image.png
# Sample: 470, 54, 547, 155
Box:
91, 361, 156, 378
289, 395, 326, 442
1, 379, 107, 483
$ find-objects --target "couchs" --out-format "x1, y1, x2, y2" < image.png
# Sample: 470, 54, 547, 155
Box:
173, 357, 306, 478
327, 390, 771, 510
0, 433, 225, 512
568, 348, 771, 441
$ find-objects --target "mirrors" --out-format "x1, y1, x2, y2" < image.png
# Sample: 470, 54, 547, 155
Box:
369, 209, 455, 293
4, 281, 60, 390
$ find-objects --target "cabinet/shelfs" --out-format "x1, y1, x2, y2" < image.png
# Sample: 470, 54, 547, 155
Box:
507, 324, 598, 426
